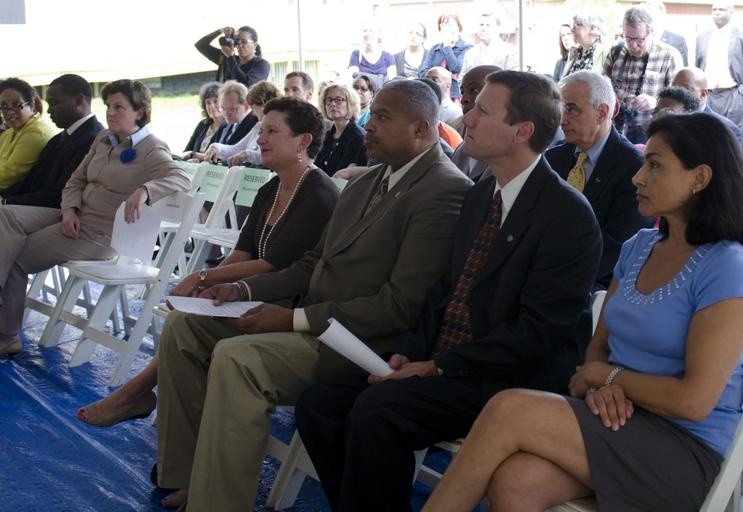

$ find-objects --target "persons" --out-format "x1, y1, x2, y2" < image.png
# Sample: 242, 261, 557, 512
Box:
552, 2, 743, 134
0, 75, 192, 352
347, 12, 520, 109
80, 64, 743, 512
195, 25, 270, 88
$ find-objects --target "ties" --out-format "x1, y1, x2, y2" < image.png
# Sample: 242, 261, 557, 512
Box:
364, 176, 390, 207
564, 151, 588, 195
431, 189, 503, 354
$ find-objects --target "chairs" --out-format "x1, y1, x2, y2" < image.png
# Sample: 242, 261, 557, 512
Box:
141, 164, 237, 299
191, 166, 278, 275
21, 253, 120, 345
137, 161, 205, 297
45, 188, 206, 389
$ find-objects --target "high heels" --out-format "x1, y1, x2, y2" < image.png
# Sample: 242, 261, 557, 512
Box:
79, 386, 158, 429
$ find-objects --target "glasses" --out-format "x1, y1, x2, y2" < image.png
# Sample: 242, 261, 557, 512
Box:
619, 33, 652, 44
324, 96, 347, 106
0, 100, 35, 115
219, 107, 239, 115
235, 39, 255, 45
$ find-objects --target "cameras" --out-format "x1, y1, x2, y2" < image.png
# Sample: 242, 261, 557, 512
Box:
218, 36, 237, 47
